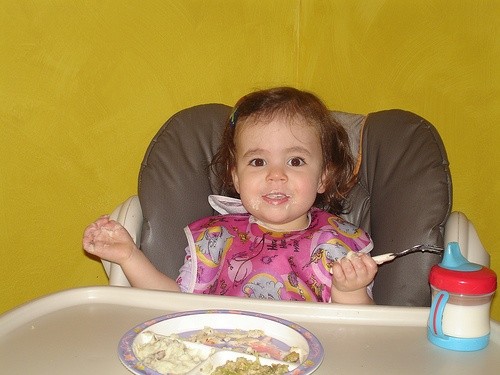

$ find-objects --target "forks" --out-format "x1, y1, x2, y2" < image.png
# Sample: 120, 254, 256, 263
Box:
329, 241, 443, 274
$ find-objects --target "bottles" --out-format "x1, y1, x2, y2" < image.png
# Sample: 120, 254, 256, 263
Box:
428, 212, 498, 351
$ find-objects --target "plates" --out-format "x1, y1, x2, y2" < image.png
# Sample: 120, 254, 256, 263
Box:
118, 310, 324, 375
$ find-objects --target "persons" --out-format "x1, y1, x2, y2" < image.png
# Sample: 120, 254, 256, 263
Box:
82, 86, 378, 306
